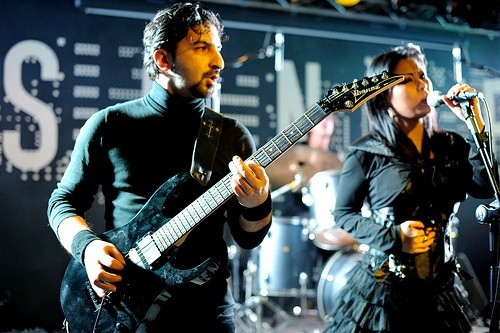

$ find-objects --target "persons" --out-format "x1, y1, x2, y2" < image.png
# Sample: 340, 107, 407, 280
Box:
318, 43, 500, 333
273, 113, 339, 220
47, 1, 272, 333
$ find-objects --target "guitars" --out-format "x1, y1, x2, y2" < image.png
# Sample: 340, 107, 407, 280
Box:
60, 70, 406, 333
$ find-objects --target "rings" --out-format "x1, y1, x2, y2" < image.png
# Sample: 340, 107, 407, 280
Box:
423, 236, 427, 242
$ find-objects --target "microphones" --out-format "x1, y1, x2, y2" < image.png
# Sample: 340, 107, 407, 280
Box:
427, 90, 482, 108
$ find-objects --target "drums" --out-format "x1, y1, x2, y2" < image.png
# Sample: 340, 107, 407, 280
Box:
317, 251, 368, 324
302, 169, 371, 251
246, 214, 336, 298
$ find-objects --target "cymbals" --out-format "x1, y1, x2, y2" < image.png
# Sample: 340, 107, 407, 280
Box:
261, 145, 344, 192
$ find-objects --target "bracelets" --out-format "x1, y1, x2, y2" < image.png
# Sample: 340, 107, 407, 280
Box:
71, 229, 101, 266
239, 189, 272, 222
